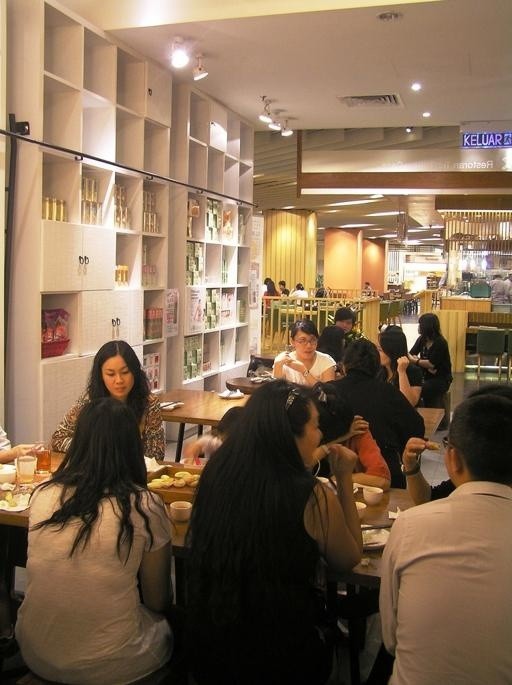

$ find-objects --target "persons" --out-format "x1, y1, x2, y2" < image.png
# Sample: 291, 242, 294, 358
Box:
0, 425, 45, 657
362, 281, 375, 296
185, 307, 452, 491
402, 385, 512, 508
14, 398, 177, 685
52, 340, 166, 460
365, 395, 512, 685
185, 380, 363, 685
264, 278, 327, 318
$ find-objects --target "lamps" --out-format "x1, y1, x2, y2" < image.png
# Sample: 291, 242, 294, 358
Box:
258, 96, 293, 138
171, 38, 209, 82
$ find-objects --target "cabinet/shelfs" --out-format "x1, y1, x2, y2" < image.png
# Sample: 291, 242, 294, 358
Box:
6, 1, 256, 446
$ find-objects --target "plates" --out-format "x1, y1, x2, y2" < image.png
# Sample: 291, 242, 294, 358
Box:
158, 402, 184, 411
218, 392, 243, 399
328, 481, 359, 495
361, 524, 391, 551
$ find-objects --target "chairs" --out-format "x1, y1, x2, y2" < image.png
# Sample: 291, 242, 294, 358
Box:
262, 292, 406, 352
477, 331, 512, 386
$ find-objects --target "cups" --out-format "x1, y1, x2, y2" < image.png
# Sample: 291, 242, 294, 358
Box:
170, 501, 193, 522
31, 445, 52, 474
14, 456, 37, 483
0, 469, 16, 484
354, 501, 367, 519
364, 486, 384, 506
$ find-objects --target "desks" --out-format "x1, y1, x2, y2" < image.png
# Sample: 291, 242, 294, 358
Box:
468, 323, 512, 335
1, 355, 445, 685
